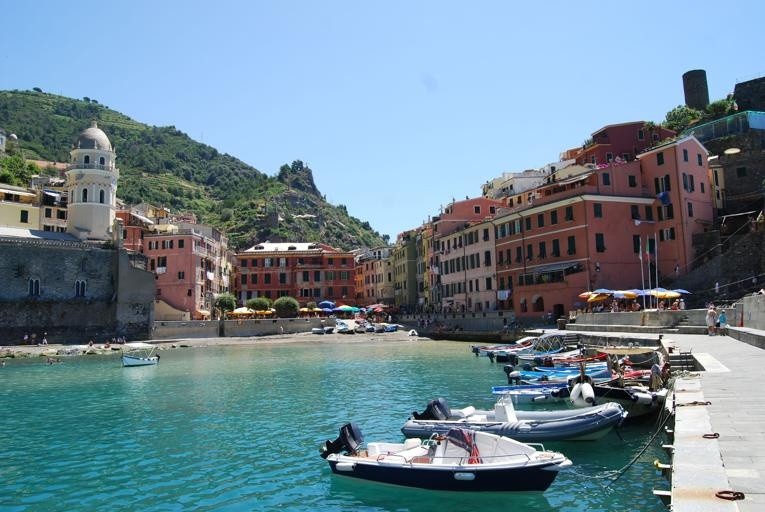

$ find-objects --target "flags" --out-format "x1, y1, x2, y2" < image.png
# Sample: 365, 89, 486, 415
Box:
654, 233, 658, 262
639, 237, 643, 261
646, 236, 650, 261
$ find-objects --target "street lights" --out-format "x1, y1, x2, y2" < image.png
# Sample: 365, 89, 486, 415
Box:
458, 224, 468, 312
486, 219, 499, 310
573, 190, 590, 291
510, 208, 527, 310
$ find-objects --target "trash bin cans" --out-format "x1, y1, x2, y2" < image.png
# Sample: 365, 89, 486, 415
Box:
557, 319, 566, 330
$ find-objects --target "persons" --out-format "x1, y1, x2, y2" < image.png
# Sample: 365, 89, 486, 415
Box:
704, 302, 709, 308
750, 217, 758, 233
21, 331, 127, 347
709, 301, 715, 308
719, 309, 727, 336
650, 364, 663, 392
661, 361, 672, 387
706, 308, 718, 335
430, 264, 435, 273
510, 314, 515, 324
547, 311, 554, 322
674, 264, 680, 279
715, 280, 721, 295
409, 302, 467, 336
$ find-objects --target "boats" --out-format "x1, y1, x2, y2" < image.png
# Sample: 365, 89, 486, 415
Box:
319, 421, 573, 492
469, 340, 648, 385
549, 361, 668, 417
491, 379, 572, 404
120, 351, 160, 366
399, 394, 629, 441
408, 329, 418, 336
310, 319, 398, 335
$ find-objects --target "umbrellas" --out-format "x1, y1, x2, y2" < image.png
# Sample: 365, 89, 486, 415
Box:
577, 287, 692, 312
223, 299, 390, 323
